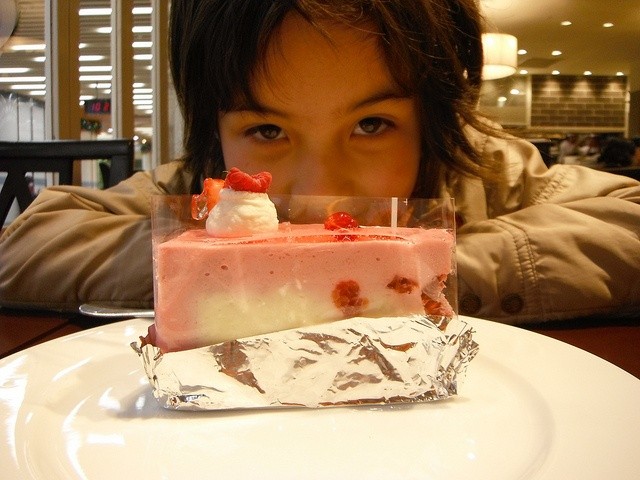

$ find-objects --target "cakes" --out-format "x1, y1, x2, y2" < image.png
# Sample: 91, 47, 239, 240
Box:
152, 166, 456, 347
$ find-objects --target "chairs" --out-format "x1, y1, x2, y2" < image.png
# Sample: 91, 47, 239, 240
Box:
0, 139, 135, 234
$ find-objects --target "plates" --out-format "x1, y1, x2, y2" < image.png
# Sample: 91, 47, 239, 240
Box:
1, 312, 639, 480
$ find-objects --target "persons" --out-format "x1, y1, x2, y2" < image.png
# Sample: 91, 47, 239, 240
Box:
1, 0, 640, 325
558, 132, 640, 170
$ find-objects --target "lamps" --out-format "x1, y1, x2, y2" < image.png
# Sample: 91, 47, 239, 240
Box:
463, 32, 519, 82
